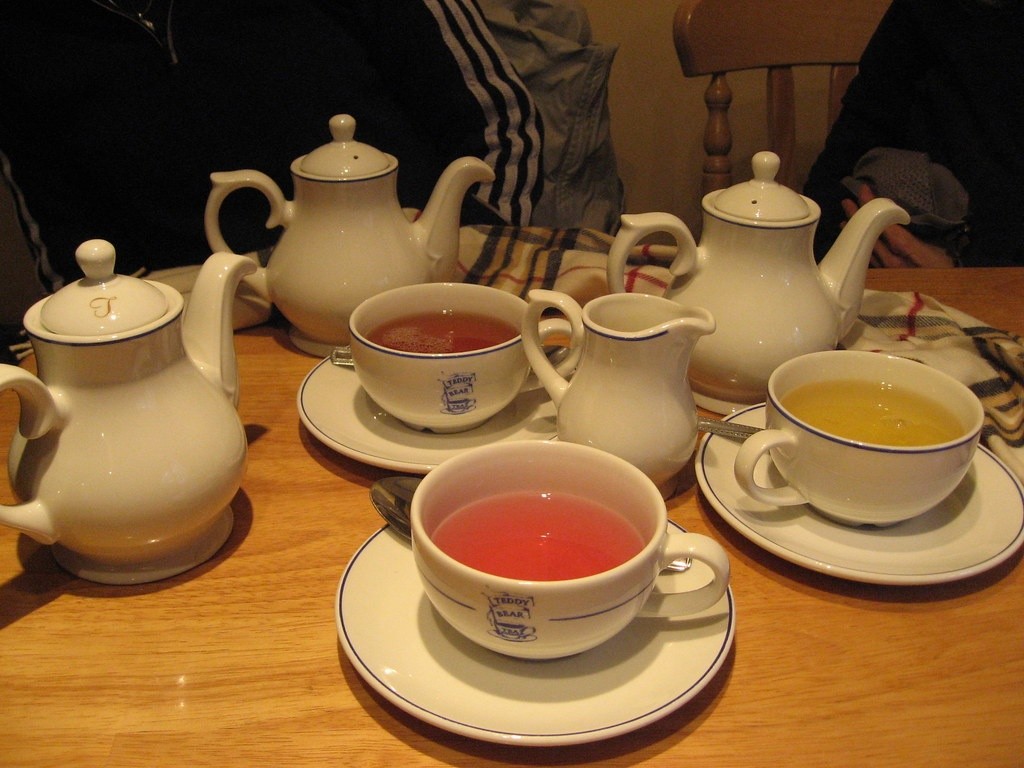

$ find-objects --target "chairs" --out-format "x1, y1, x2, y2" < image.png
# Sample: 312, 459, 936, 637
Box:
672, 0, 893, 196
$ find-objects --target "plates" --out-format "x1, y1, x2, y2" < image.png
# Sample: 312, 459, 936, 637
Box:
694, 401, 1024, 586
335, 517, 736, 747
296, 344, 572, 474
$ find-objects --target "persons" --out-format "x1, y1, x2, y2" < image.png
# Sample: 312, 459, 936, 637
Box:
0, 0, 543, 296
802, 0, 1024, 269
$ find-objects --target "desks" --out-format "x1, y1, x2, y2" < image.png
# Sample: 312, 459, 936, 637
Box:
0, 224, 1024, 768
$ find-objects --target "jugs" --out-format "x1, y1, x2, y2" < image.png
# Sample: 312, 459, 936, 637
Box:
521, 289, 717, 501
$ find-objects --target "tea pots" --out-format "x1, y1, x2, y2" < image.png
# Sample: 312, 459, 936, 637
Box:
0, 239, 259, 586
205, 114, 495, 359
605, 150, 911, 416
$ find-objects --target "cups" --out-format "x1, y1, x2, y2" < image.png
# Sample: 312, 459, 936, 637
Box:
734, 350, 985, 528
410, 440, 730, 660
350, 282, 582, 434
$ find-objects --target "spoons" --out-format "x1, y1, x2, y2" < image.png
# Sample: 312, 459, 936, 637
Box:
369, 476, 692, 573
